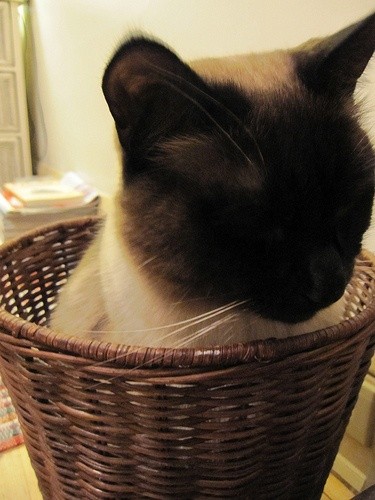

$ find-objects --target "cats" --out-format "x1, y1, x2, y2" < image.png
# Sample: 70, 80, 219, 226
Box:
48, 11, 375, 390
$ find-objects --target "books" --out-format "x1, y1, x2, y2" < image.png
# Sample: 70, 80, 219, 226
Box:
0, 169, 103, 455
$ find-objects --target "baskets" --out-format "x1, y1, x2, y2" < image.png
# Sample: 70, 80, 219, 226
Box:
1, 216, 375, 500
